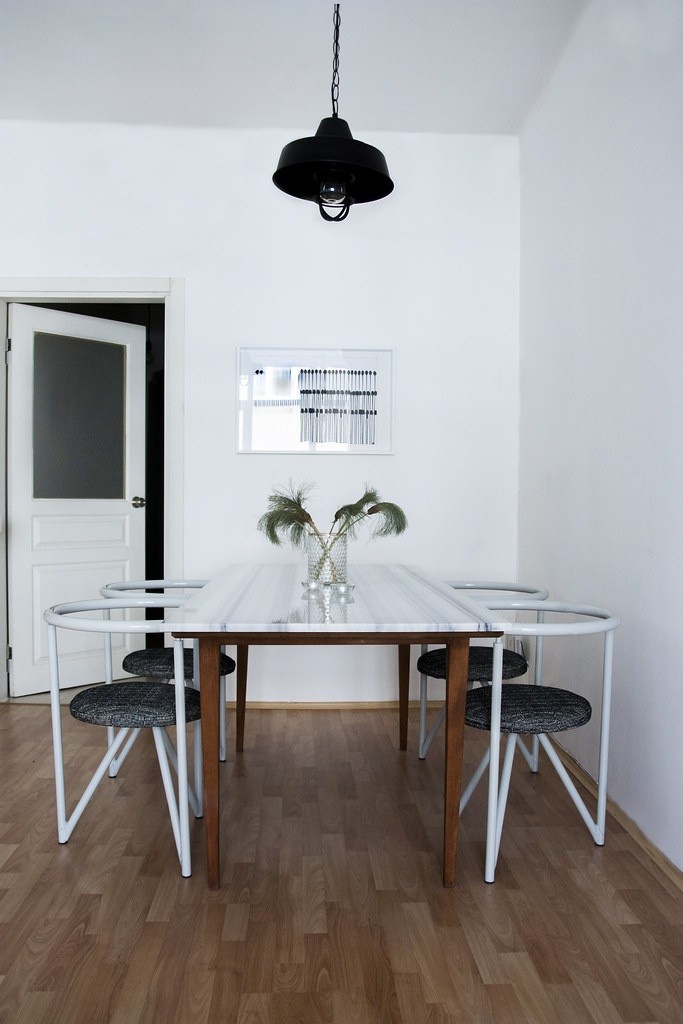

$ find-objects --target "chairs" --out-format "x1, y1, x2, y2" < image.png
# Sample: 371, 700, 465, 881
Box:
98, 582, 234, 778
455, 600, 619, 884
418, 577, 545, 774
41, 593, 209, 878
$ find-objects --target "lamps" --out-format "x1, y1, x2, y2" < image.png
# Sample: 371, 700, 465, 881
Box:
272, 3, 394, 222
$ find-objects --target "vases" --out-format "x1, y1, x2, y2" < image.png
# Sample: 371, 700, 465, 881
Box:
307, 532, 347, 586
307, 586, 349, 624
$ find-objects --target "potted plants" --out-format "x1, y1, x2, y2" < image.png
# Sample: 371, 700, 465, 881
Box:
259, 486, 408, 586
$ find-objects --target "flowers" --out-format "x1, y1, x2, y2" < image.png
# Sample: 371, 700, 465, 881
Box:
160, 555, 503, 888
